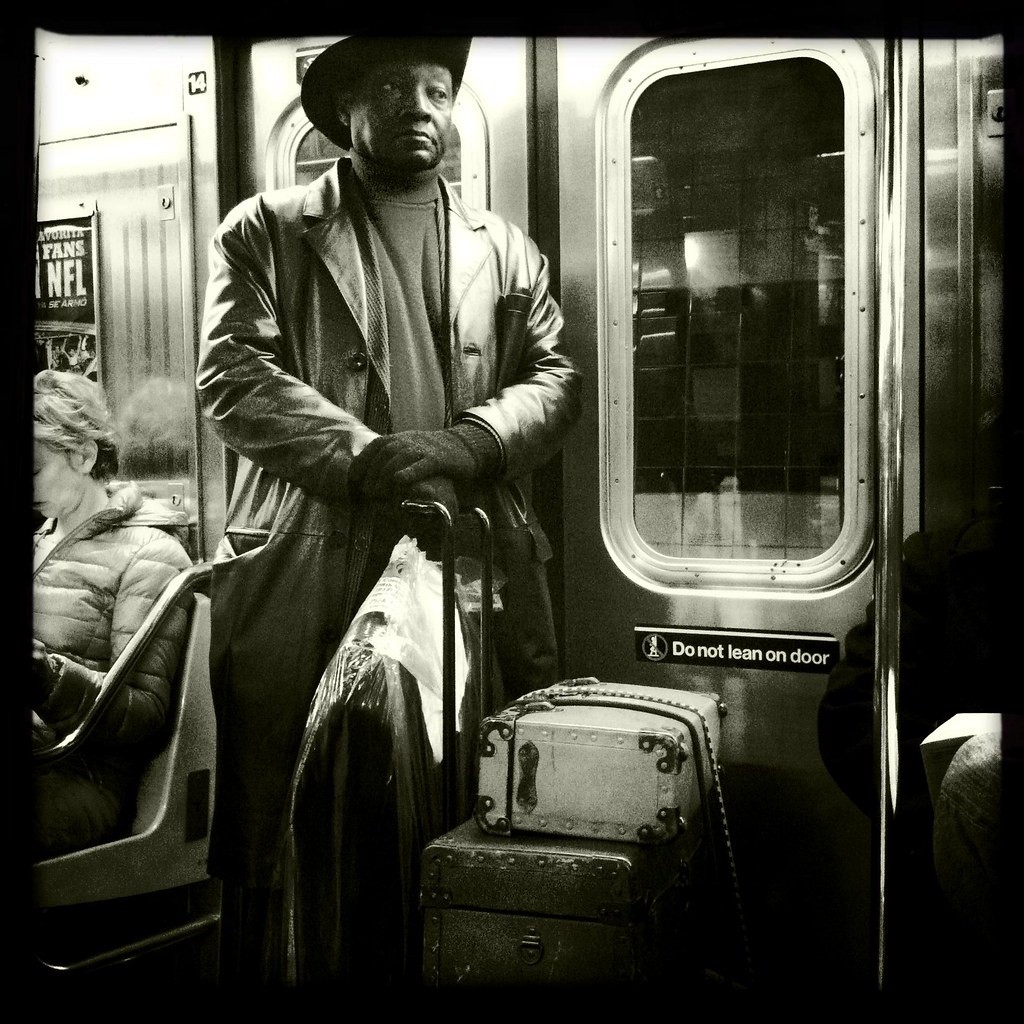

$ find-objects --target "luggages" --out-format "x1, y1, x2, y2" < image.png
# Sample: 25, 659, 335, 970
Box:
416, 827, 668, 1014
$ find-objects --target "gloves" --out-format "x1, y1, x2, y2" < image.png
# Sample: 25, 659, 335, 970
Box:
354, 423, 497, 522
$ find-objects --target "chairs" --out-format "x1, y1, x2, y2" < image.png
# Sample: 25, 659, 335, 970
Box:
16, 591, 219, 907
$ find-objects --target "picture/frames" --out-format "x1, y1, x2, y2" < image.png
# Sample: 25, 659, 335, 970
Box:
32, 198, 104, 387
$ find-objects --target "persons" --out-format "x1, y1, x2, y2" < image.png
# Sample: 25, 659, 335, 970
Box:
199, 35, 585, 1023
33, 370, 196, 865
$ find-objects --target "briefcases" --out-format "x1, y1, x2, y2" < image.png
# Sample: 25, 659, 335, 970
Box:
465, 664, 734, 843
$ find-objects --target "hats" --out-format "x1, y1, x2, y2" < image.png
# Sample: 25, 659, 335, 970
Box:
302, 33, 473, 149
